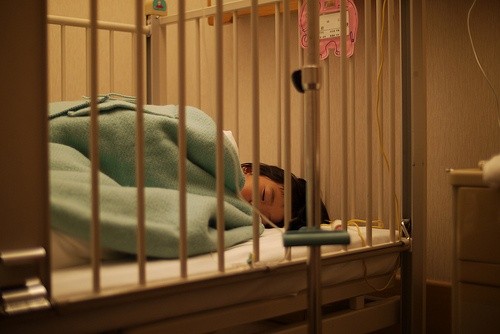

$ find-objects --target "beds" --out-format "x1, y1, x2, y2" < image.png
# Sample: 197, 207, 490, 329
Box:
0, 1, 425, 334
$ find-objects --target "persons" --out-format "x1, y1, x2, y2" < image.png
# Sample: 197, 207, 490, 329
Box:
237, 160, 331, 230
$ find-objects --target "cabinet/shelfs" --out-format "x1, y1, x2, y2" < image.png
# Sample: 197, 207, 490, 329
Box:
450, 169, 500, 334
